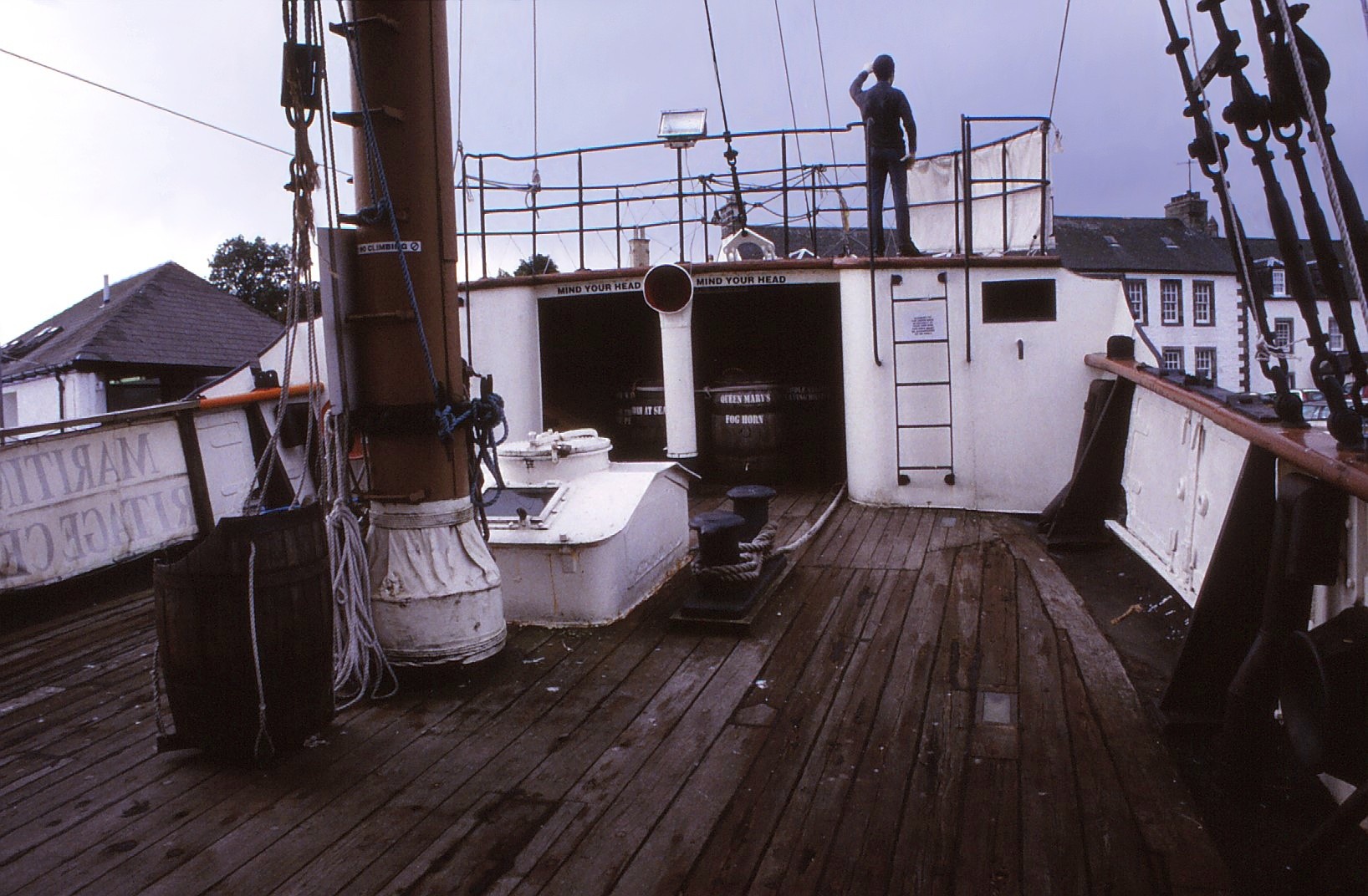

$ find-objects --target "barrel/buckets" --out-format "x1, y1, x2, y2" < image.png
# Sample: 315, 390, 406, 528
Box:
617, 381, 711, 487
707, 380, 783, 485
780, 381, 842, 487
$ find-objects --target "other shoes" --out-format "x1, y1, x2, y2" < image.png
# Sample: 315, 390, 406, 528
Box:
900, 246, 921, 257
873, 251, 884, 257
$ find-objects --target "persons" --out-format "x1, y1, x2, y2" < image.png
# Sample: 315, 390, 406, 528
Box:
849, 54, 934, 258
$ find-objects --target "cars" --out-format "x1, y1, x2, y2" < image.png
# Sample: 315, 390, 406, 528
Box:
1238, 381, 1368, 403
